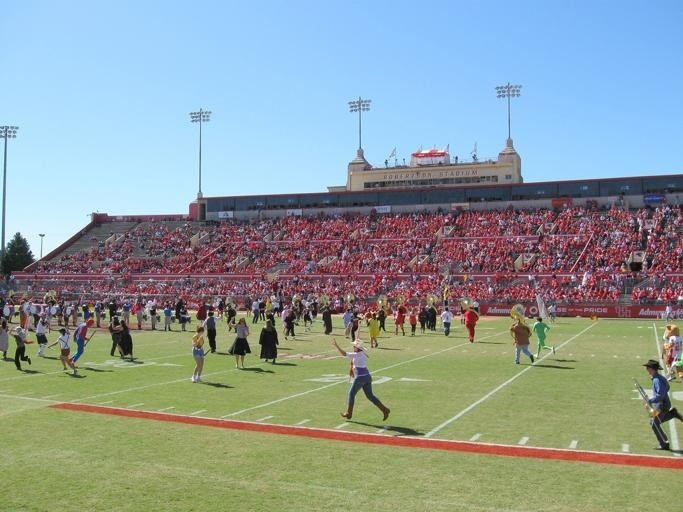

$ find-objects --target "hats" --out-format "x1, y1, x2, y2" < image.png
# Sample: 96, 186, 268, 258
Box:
643, 360, 663, 370
351, 339, 364, 350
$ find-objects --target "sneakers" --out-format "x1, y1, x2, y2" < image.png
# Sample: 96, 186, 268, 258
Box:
192, 377, 200, 382
656, 444, 669, 449
672, 408, 682, 421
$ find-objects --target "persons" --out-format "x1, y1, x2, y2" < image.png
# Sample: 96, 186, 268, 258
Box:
643, 359, 682, 451
332, 337, 391, 421
1, 191, 683, 383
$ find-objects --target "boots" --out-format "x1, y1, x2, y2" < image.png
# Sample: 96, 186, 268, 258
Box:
340, 405, 353, 419
378, 402, 390, 420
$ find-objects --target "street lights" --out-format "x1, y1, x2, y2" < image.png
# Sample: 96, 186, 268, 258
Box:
347, 95, 372, 157
495, 79, 523, 149
188, 107, 212, 201
0, 125, 18, 252
37, 232, 44, 259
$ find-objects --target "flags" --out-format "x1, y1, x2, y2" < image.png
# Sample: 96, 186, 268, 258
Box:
430, 145, 436, 154
443, 144, 449, 153
416, 144, 422, 155
470, 142, 477, 154
389, 145, 396, 158
